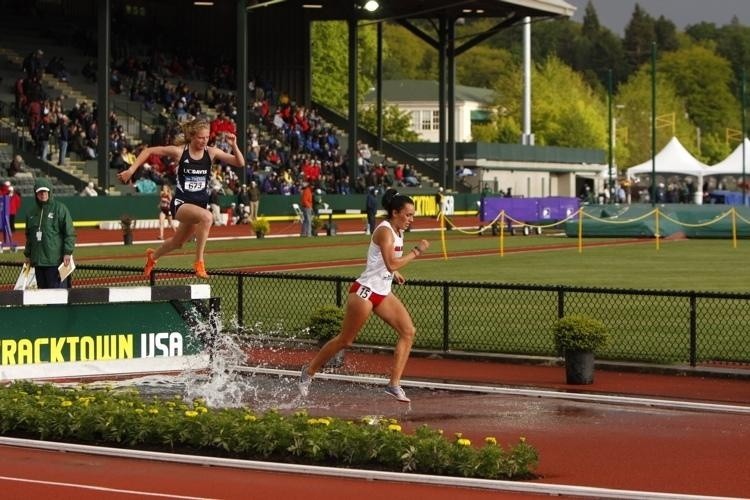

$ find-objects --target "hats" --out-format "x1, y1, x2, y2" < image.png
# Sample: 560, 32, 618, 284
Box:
36, 186, 49, 193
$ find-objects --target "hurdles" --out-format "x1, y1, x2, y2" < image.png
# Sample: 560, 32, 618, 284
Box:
0, 284, 220, 366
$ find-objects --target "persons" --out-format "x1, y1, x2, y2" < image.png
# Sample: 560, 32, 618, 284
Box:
481, 183, 513, 198
0, 48, 423, 288
578, 172, 750, 204
434, 185, 444, 223
298, 189, 432, 404
460, 176, 467, 185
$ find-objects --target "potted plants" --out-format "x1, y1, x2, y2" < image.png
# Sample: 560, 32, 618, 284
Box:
310, 304, 351, 366
252, 220, 271, 240
550, 314, 609, 385
311, 218, 324, 236
120, 215, 134, 245
445, 217, 454, 231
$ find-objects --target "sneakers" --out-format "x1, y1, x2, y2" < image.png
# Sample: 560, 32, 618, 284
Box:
297, 365, 312, 397
145, 248, 157, 276
384, 385, 411, 402
192, 260, 209, 279
